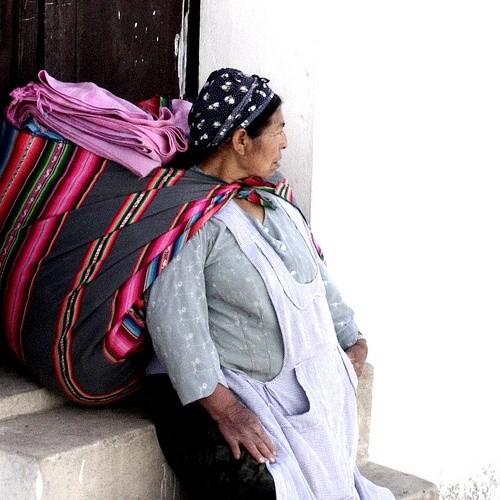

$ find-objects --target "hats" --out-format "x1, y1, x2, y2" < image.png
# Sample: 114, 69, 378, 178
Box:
185, 68, 275, 153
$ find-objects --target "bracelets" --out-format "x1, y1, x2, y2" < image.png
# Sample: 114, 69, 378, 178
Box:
357, 332, 367, 341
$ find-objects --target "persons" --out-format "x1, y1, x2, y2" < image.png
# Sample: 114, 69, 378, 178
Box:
138, 67, 368, 499
